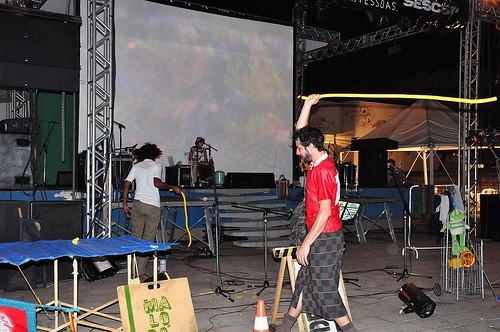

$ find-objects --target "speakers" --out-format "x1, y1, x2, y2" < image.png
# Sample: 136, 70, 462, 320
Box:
351, 146, 387, 188
222, 172, 277, 188
0, 200, 30, 289
0, 3, 83, 94
480, 194, 500, 240
30, 200, 84, 283
56, 171, 78, 188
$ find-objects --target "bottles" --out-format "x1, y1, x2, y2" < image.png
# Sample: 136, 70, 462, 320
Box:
159, 258, 167, 273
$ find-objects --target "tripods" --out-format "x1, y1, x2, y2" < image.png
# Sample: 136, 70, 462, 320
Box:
385, 162, 432, 282
191, 177, 294, 302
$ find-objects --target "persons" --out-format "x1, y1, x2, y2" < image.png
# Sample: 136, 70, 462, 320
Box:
123, 143, 181, 281
188, 137, 208, 161
270, 94, 356, 332
387, 159, 406, 187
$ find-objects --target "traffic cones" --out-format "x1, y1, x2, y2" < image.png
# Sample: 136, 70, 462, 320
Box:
252, 300, 273, 332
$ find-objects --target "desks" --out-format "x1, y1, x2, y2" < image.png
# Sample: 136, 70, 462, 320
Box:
339, 197, 399, 245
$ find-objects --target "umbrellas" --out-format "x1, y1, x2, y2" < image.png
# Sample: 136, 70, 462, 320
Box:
339, 99, 494, 183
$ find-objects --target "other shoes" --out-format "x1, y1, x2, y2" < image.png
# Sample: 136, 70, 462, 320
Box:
132, 274, 150, 280
